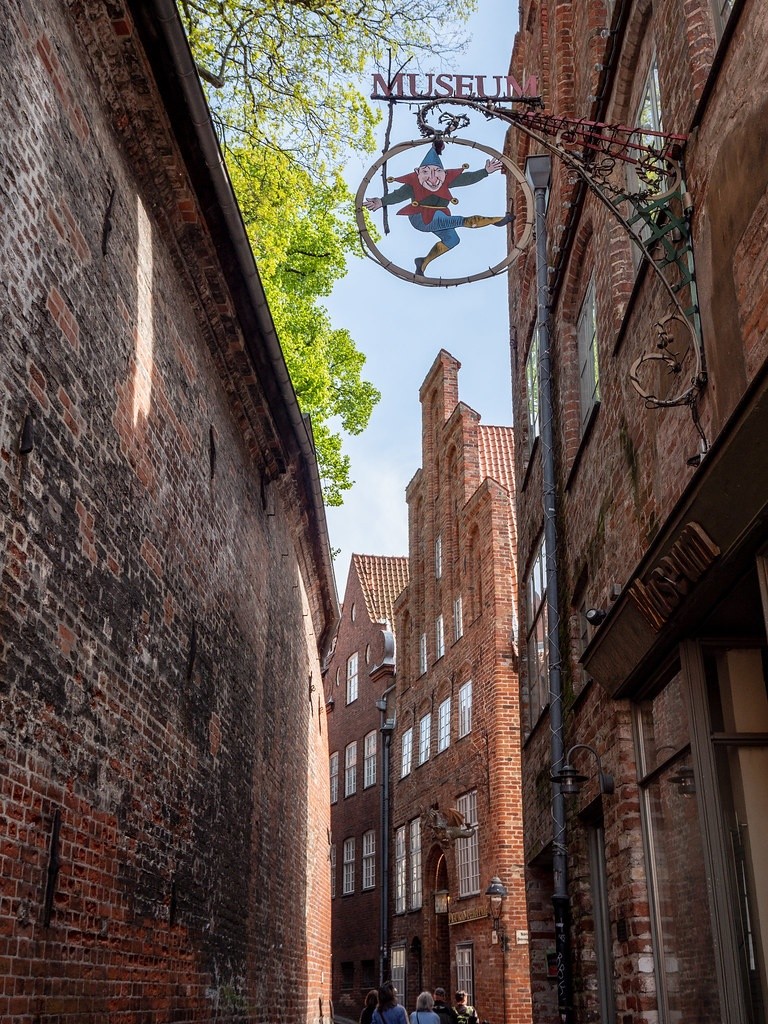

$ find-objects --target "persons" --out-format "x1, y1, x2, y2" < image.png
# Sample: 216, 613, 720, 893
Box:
359, 980, 479, 1024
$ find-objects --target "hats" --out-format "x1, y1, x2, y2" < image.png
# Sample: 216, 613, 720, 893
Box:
435, 987, 445, 995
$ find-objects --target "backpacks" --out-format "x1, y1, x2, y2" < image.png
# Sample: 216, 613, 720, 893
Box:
452, 1007, 473, 1024
